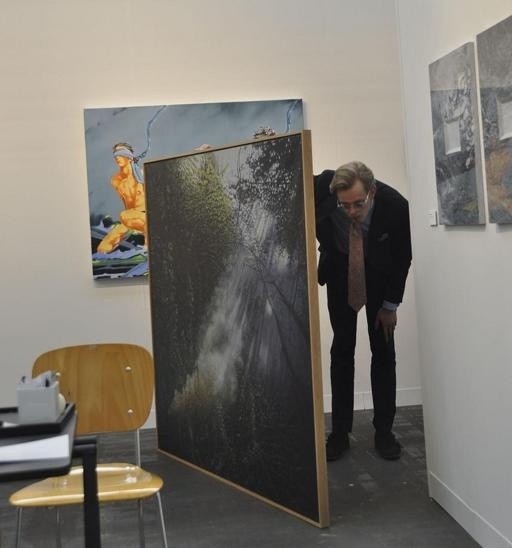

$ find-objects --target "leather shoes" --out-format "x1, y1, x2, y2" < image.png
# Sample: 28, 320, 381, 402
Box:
326, 432, 348, 462
374, 427, 402, 462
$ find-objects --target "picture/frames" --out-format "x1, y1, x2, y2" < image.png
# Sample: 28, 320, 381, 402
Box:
475, 15, 512, 225
143, 129, 328, 529
83, 98, 305, 282
428, 42, 487, 226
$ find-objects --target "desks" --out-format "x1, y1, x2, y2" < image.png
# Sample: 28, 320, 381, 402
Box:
0, 404, 101, 548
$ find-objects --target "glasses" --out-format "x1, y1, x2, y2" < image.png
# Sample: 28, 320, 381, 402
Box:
335, 193, 370, 211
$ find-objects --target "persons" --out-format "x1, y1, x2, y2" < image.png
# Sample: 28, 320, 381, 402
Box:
192, 142, 416, 462
94, 141, 145, 255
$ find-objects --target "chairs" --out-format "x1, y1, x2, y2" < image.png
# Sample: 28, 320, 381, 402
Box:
9, 344, 168, 548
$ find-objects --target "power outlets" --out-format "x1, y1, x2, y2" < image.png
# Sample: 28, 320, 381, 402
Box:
427, 210, 437, 226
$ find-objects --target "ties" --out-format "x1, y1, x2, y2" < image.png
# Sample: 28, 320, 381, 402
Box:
349, 218, 365, 313
